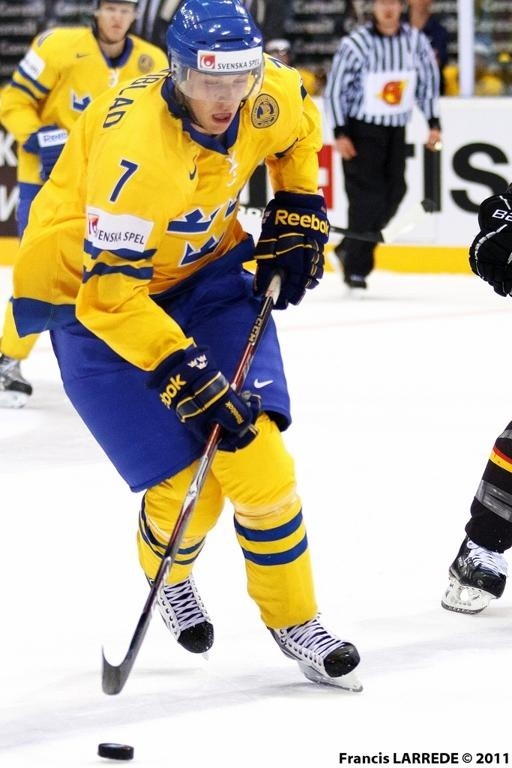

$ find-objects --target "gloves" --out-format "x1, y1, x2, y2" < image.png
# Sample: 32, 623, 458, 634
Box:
23, 125, 67, 182
151, 346, 260, 453
251, 191, 329, 311
471, 187, 512, 296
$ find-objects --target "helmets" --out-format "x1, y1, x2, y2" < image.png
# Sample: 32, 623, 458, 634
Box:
168, 0, 265, 103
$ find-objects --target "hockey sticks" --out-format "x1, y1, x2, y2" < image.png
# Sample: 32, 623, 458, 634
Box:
237, 201, 433, 243
101, 274, 282, 694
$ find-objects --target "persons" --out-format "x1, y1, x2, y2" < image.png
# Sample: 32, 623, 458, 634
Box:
398, 0, 511, 210
11, 0, 358, 681
326, 0, 440, 288
244, 39, 316, 216
0, 0, 169, 394
449, 182, 512, 600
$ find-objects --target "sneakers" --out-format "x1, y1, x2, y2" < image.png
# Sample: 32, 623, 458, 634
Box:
264, 616, 362, 679
145, 574, 215, 656
333, 237, 371, 292
447, 535, 508, 600
1, 352, 33, 394
